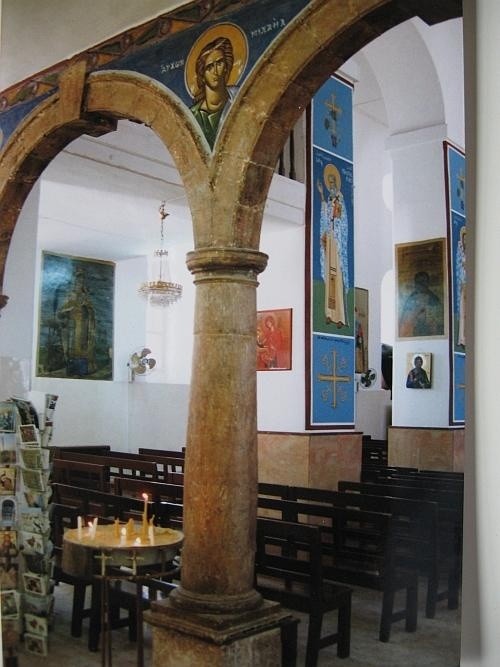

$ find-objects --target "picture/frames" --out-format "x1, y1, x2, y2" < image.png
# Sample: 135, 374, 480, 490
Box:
393, 237, 450, 340
254, 307, 293, 371
353, 286, 369, 373
36, 248, 117, 380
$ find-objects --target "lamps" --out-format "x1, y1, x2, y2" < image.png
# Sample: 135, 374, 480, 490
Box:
137, 202, 183, 307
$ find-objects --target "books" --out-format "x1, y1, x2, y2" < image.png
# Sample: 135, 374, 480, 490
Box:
0, 392, 59, 658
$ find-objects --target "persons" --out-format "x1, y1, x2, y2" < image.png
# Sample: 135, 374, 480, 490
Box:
315, 173, 353, 329
321, 190, 343, 252
185, 36, 243, 149
396, 270, 443, 336
406, 355, 432, 389
54, 267, 98, 376
255, 315, 284, 369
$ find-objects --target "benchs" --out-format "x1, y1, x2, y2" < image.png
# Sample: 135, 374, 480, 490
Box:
48, 445, 185, 653
361, 439, 389, 470
255, 467, 461, 667
